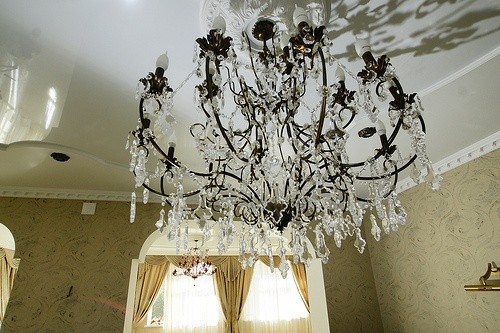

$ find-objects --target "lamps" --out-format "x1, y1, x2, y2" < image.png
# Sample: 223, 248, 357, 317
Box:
125, 2, 443, 280
